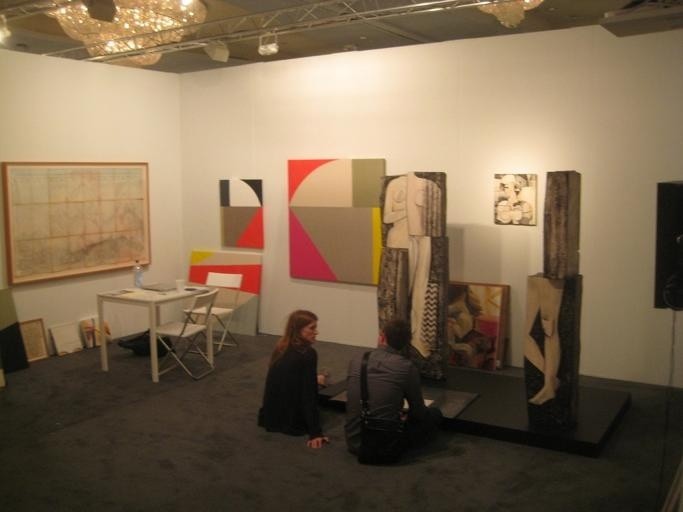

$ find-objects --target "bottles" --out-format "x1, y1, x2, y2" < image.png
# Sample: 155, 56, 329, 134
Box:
132, 259, 143, 288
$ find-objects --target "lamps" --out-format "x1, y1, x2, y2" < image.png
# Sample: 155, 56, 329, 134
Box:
476, 0, 542, 27
257, 32, 279, 54
206, 41, 231, 62
43, 0, 206, 65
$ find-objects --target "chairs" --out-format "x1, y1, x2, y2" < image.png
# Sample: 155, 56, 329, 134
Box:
154, 288, 215, 379
184, 271, 244, 353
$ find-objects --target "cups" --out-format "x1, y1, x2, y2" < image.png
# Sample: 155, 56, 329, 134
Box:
176, 279, 185, 293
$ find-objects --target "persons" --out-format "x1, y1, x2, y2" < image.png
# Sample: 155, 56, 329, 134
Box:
524, 276, 566, 406
383, 172, 441, 359
344, 318, 444, 456
447, 284, 496, 372
261, 308, 330, 449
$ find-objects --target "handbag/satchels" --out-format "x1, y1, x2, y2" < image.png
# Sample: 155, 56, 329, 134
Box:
359, 417, 418, 466
118, 335, 171, 357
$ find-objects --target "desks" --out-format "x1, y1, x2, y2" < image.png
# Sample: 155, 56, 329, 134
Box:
95, 283, 216, 383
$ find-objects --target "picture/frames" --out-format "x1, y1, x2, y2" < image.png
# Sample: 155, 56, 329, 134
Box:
0, 160, 151, 284
48, 321, 84, 355
19, 318, 48, 362
79, 316, 111, 349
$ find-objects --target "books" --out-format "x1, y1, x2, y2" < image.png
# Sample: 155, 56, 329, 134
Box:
141, 282, 177, 292
